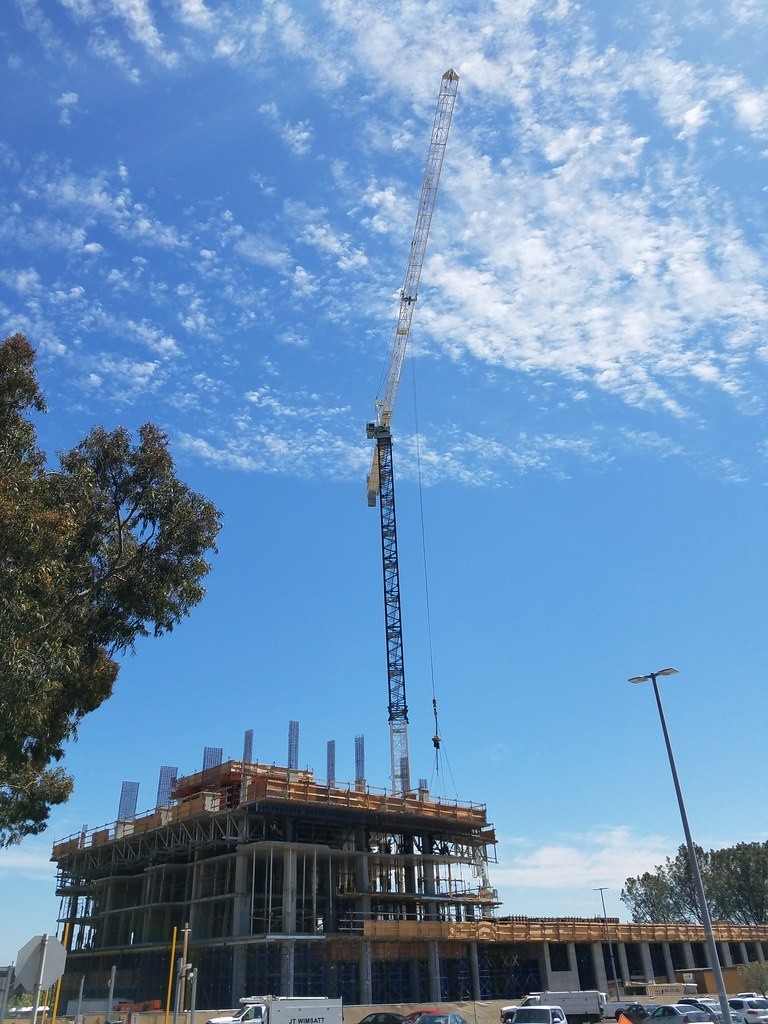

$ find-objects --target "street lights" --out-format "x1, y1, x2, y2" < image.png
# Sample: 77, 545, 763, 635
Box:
628, 668, 735, 1024
180, 923, 192, 1014
592, 886, 621, 1002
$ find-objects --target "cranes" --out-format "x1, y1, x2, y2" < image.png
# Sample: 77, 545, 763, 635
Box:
364, 51, 470, 797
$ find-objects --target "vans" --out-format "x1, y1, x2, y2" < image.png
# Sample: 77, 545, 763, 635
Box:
505, 1005, 569, 1024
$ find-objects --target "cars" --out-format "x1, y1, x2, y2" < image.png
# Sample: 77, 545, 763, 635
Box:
499, 989, 768, 1024
356, 1009, 468, 1024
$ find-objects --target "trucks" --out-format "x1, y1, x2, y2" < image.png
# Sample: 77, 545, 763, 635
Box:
205, 993, 345, 1024
